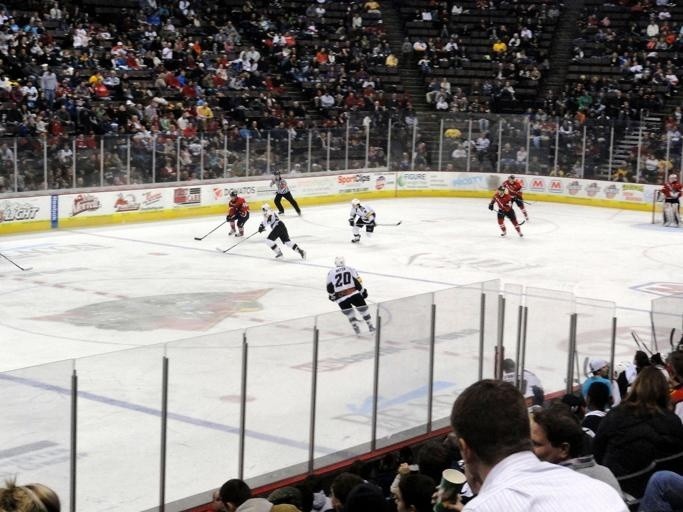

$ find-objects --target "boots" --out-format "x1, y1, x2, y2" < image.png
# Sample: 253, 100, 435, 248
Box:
351, 235, 360, 242
228, 225, 235, 235
297, 248, 304, 258
353, 324, 360, 334
367, 321, 376, 331
235, 230, 244, 237
275, 247, 282, 257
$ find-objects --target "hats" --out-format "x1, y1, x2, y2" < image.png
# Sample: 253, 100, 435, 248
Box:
585, 358, 608, 375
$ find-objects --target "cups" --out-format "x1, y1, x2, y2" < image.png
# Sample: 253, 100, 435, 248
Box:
433, 469, 467, 512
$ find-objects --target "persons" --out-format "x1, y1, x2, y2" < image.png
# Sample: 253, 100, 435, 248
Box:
658, 173, 682, 228
226, 191, 249, 238
326, 257, 376, 337
269, 169, 301, 217
348, 198, 375, 244
220, 350, 683, 511
258, 202, 303, 260
0, 2, 682, 192
487, 186, 523, 239
0, 477, 61, 511
499, 175, 528, 221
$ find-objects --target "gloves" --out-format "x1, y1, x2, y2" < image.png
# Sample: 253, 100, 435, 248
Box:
226, 215, 230, 221
489, 203, 493, 211
258, 224, 263, 232
362, 289, 367, 298
349, 219, 353, 226
370, 220, 376, 227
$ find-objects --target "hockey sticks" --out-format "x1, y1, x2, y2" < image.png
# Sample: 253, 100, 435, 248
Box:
355, 221, 402, 226
492, 210, 525, 225
0, 253, 34, 271
216, 230, 263, 252
194, 221, 226, 240
516, 198, 537, 206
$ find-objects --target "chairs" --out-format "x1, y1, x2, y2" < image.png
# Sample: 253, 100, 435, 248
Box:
613, 453, 682, 511
0, 2, 681, 184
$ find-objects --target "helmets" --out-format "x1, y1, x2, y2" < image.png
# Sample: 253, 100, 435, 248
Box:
229, 192, 236, 196
351, 199, 360, 209
668, 174, 677, 182
508, 175, 516, 182
261, 204, 270, 213
497, 186, 505, 190
274, 171, 280, 175
334, 256, 344, 267
